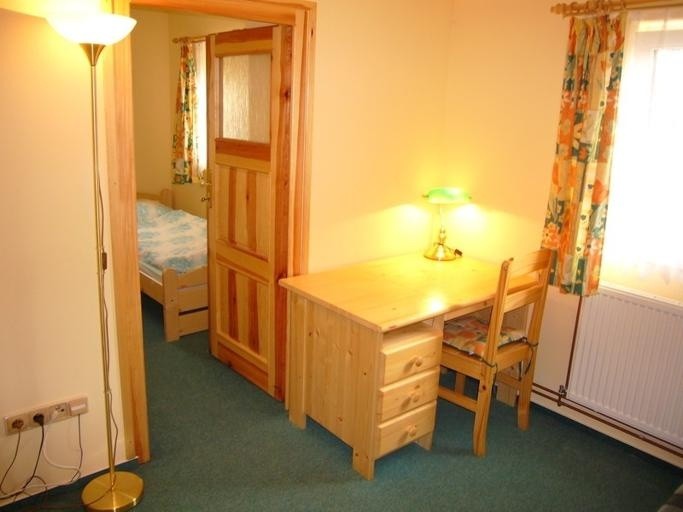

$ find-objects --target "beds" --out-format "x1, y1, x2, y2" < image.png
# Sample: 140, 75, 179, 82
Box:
130, 185, 214, 342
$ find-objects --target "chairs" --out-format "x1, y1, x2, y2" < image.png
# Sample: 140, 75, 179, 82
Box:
441, 248, 556, 458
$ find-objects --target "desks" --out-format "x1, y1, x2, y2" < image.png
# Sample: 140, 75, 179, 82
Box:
271, 253, 539, 480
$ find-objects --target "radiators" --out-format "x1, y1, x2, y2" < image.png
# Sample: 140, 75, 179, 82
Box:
564, 275, 683, 452
